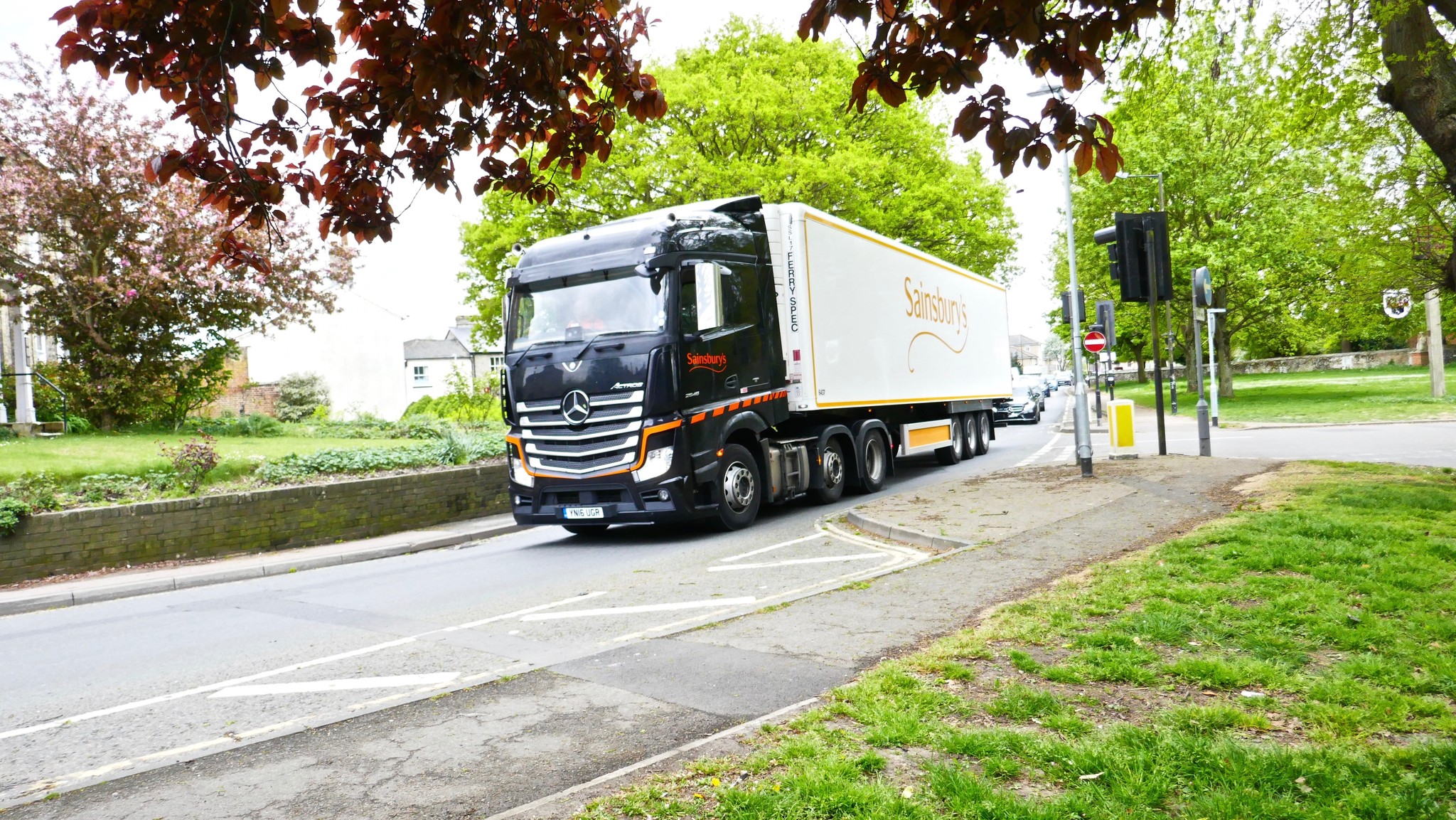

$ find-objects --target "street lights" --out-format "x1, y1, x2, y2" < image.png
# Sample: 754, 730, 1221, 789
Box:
1116, 170, 1177, 415
1024, 82, 1094, 480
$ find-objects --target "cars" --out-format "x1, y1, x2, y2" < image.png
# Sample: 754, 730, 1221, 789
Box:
991, 368, 1075, 427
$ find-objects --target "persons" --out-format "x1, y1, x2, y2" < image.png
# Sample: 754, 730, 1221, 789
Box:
566, 299, 604, 330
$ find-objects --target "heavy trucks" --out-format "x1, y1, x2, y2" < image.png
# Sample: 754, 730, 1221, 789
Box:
493, 189, 1016, 543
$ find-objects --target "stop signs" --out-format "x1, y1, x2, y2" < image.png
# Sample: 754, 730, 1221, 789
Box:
1083, 331, 1106, 353
